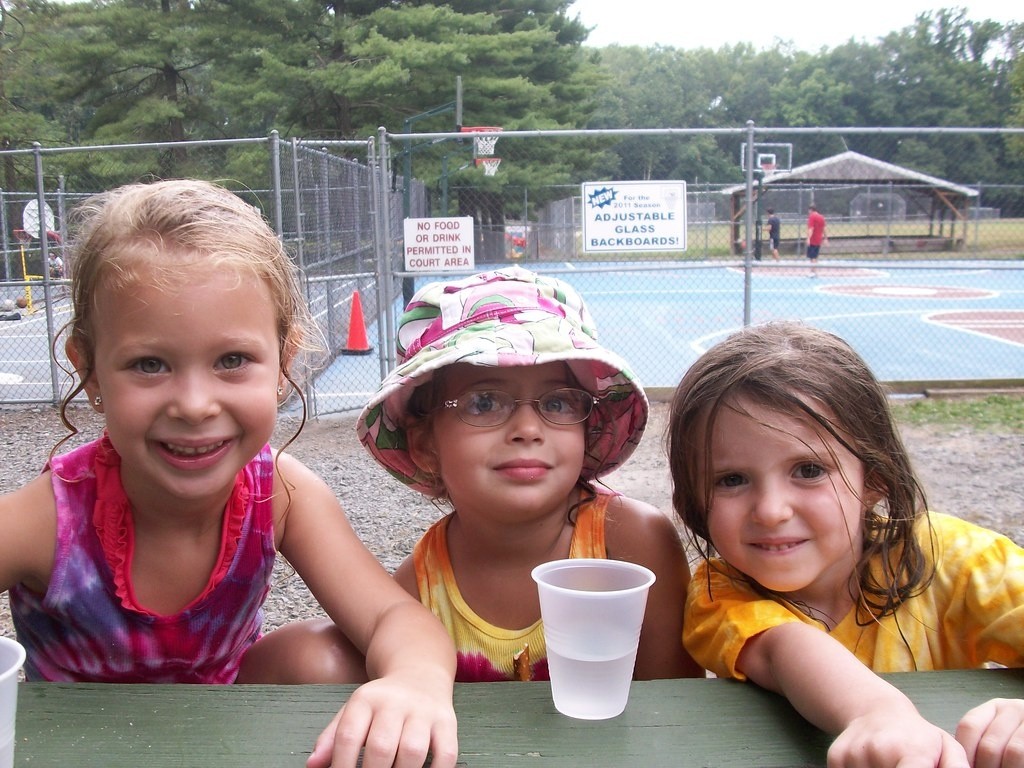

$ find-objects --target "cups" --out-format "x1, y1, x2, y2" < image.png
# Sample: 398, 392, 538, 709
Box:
531, 558, 656, 722
0, 636, 27, 768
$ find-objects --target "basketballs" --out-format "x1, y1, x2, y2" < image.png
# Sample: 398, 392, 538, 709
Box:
16, 296, 27, 308
3, 299, 14, 311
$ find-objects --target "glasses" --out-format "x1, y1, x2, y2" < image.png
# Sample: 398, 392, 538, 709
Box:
420, 388, 600, 425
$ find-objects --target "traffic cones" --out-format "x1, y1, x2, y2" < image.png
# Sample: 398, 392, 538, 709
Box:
337, 291, 375, 356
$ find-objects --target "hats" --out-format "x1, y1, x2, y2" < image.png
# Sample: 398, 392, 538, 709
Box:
355, 266, 650, 498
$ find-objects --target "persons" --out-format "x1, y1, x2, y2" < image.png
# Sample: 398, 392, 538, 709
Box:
806, 204, 829, 279
760, 209, 781, 263
669, 323, 1024, 768
48, 253, 64, 279
357, 266, 706, 682
0, 179, 459, 768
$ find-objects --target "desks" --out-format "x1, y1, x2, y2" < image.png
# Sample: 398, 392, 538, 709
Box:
0, 666, 1024, 768
